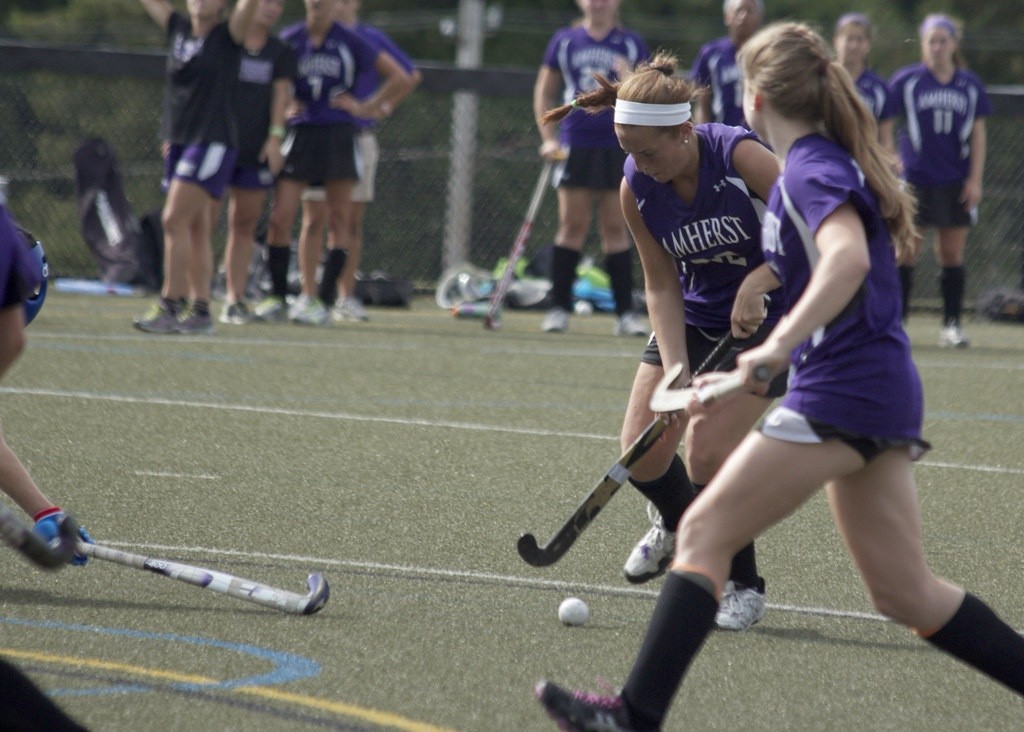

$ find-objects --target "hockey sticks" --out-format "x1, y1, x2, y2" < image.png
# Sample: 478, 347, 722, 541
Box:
76, 540, 331, 615
649, 361, 770, 413
484, 163, 555, 330
518, 290, 772, 567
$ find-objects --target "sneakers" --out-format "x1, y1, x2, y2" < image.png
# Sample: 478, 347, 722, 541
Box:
173, 309, 217, 335
533, 677, 634, 732
937, 316, 970, 348
333, 297, 370, 324
613, 312, 649, 337
131, 297, 181, 335
289, 293, 328, 324
710, 575, 767, 633
218, 301, 258, 326
254, 296, 290, 322
622, 499, 677, 585
541, 307, 570, 333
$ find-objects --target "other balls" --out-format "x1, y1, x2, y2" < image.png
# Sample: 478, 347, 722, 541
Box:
574, 300, 594, 317
558, 597, 590, 626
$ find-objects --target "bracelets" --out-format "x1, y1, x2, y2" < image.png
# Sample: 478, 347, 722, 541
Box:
269, 125, 287, 139
371, 96, 393, 117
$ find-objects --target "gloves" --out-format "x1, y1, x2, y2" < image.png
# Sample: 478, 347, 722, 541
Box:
29, 505, 96, 567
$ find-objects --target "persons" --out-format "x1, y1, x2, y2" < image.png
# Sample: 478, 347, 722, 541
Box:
829, 8, 891, 155
688, 1, 765, 135
131, 0, 423, 334
534, 0, 652, 338
0, 197, 93, 568
887, 11, 992, 348
534, 20, 1024, 732
540, 43, 790, 632
70, 129, 168, 292
